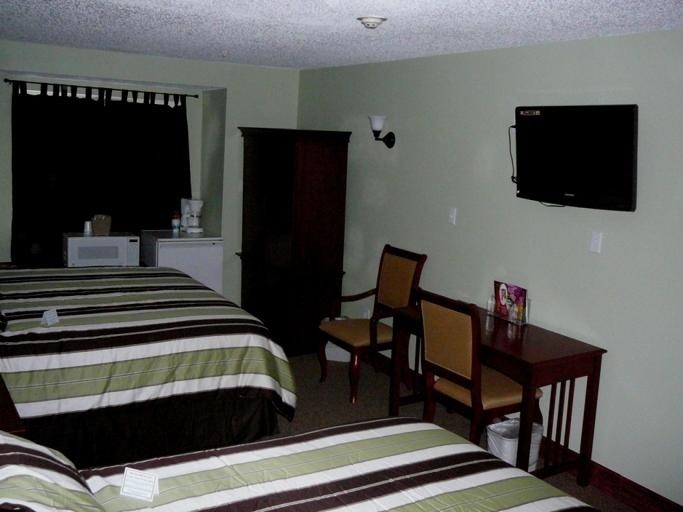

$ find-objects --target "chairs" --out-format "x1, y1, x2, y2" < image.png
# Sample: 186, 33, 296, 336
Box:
389, 286, 543, 445
316, 244, 427, 404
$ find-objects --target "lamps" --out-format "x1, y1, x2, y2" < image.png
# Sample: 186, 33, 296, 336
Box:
369, 115, 395, 148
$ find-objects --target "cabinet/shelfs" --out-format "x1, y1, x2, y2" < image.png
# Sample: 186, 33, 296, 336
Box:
236, 127, 352, 357
141, 229, 224, 296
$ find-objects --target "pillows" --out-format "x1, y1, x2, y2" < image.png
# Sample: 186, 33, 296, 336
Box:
0, 429, 105, 511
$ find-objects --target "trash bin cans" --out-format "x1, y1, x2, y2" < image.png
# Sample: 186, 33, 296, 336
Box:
487, 419, 543, 472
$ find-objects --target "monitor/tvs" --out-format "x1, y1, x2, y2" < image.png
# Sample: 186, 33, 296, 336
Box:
515, 103, 639, 212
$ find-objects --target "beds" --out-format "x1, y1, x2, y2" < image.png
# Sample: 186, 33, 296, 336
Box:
0, 267, 297, 469
78, 417, 601, 511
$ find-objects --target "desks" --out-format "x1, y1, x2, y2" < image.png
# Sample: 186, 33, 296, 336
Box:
388, 299, 607, 488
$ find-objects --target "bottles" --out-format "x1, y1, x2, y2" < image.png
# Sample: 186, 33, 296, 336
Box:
172, 219, 180, 233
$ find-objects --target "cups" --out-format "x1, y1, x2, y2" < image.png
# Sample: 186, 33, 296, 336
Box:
84, 221, 91, 235
